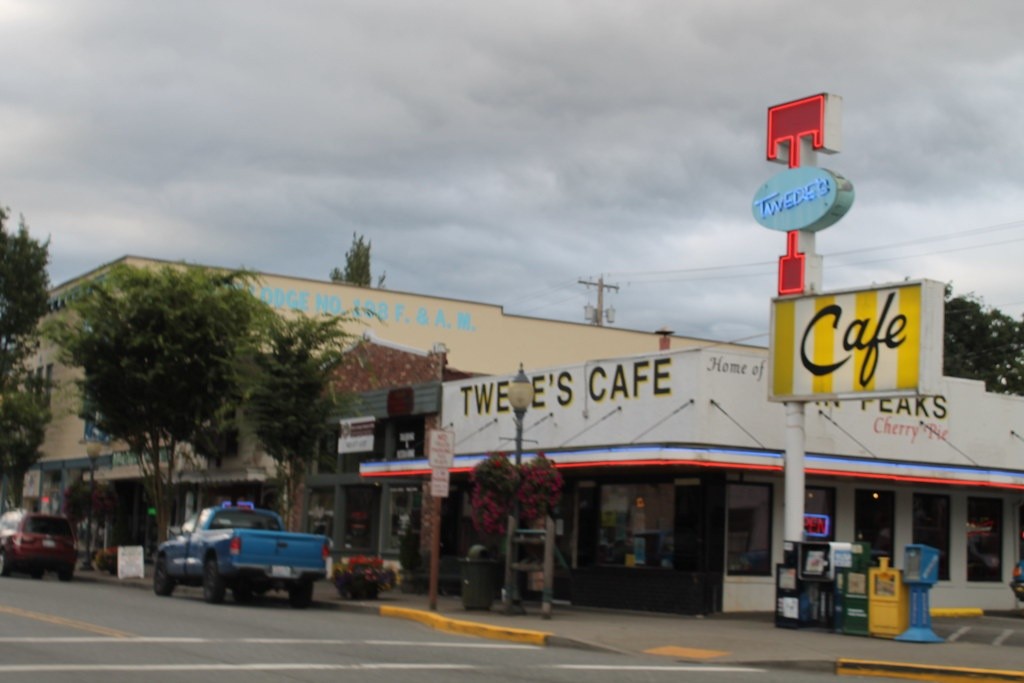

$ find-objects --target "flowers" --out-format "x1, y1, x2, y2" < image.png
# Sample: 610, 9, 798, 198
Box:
97, 546, 118, 570
465, 445, 565, 535
332, 553, 391, 595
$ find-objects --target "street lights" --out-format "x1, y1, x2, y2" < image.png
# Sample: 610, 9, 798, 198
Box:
78, 434, 97, 572
497, 363, 534, 615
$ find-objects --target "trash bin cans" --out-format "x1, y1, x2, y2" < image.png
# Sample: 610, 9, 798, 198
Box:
460, 545, 499, 610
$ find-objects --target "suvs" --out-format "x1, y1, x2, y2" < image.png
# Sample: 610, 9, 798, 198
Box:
1, 510, 77, 582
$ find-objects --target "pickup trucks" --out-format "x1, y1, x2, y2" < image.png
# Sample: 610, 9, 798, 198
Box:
160, 502, 333, 606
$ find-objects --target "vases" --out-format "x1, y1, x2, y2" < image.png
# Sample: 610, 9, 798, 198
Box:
109, 568, 117, 573
351, 578, 376, 599
